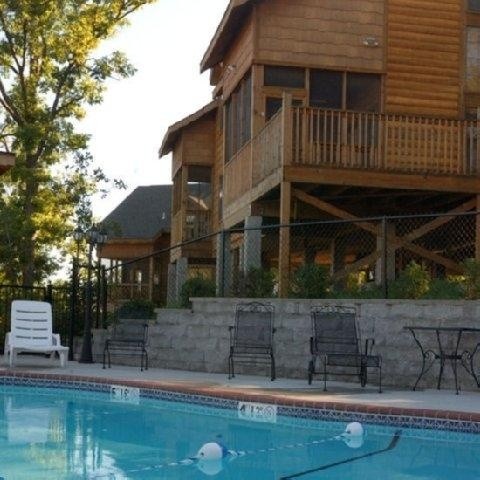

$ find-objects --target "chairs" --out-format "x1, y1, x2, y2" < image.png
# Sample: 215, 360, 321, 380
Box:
102, 302, 149, 372
226, 300, 278, 382
307, 305, 384, 394
4, 299, 69, 371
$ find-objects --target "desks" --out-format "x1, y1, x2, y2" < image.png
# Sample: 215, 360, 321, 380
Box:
401, 325, 480, 395
